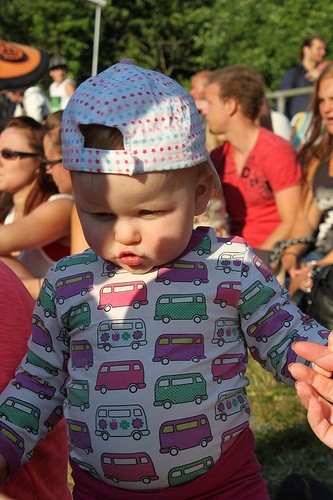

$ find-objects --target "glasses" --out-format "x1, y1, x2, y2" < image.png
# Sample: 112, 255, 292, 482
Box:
0, 148, 39, 159
40, 159, 62, 168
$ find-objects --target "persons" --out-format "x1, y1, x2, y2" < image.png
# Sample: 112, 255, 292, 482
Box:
0, 38, 333, 500
0, 59, 333, 500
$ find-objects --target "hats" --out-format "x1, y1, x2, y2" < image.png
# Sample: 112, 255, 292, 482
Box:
48, 57, 68, 70
61, 59, 222, 190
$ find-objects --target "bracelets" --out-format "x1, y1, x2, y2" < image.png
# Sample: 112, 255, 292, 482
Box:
283, 251, 298, 259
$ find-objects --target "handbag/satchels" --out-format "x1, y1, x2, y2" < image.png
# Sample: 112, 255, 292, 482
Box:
269, 237, 333, 331
